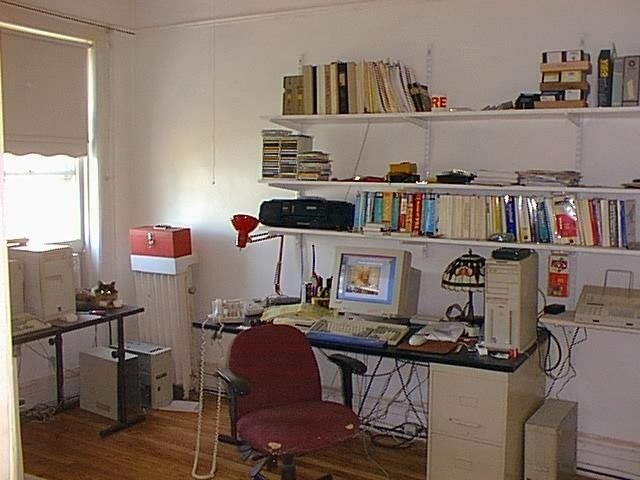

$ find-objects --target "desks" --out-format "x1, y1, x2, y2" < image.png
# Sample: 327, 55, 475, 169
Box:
190, 299, 548, 480
12, 297, 146, 437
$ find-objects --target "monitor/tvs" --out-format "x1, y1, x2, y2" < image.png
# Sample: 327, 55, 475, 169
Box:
329, 245, 421, 317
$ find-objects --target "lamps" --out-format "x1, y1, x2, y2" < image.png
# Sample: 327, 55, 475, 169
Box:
229, 213, 284, 302
438, 247, 487, 327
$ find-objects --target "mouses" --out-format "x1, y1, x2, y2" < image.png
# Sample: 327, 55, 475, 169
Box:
409, 333, 427, 346
57, 311, 78, 323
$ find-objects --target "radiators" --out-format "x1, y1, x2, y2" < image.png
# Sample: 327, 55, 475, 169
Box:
133, 271, 197, 399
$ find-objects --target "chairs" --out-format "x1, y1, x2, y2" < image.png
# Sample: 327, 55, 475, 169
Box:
212, 323, 367, 480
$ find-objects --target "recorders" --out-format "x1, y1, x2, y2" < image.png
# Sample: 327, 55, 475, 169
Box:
259, 196, 356, 234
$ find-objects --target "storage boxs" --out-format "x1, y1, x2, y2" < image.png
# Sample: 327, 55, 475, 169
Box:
129, 255, 199, 274
129, 224, 192, 256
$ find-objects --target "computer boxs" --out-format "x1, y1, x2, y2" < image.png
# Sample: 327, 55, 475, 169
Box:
109, 345, 173, 410
11, 246, 76, 322
483, 253, 538, 354
80, 348, 142, 420
524, 399, 578, 480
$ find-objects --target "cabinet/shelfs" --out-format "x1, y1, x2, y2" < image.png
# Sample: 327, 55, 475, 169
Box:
257, 39, 640, 255
425, 361, 510, 478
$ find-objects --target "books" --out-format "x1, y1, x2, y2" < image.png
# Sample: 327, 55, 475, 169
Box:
257, 129, 333, 183
344, 192, 640, 248
469, 169, 518, 189
593, 49, 639, 107
520, 170, 584, 188
282, 60, 430, 114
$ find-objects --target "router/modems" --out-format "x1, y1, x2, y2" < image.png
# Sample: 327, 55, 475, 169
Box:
410, 314, 444, 325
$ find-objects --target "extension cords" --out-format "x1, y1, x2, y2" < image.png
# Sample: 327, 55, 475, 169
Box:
403, 423, 418, 436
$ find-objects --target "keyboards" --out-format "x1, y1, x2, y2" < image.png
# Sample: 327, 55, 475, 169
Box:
308, 316, 408, 346
12, 314, 52, 337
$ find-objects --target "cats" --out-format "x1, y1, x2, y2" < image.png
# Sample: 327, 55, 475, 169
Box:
75, 279, 118, 309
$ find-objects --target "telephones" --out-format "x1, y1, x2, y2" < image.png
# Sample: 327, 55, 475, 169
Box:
206, 296, 247, 324
